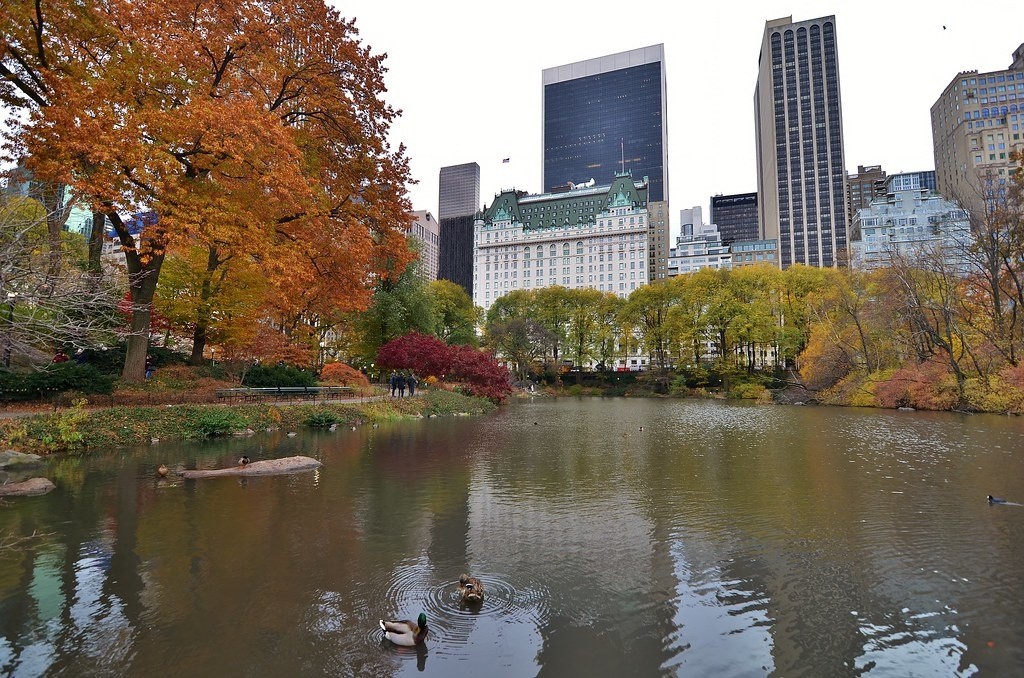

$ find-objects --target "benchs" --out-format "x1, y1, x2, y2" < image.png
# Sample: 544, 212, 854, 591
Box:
216, 387, 354, 406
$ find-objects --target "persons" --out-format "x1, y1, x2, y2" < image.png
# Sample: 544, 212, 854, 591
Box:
390, 369, 416, 397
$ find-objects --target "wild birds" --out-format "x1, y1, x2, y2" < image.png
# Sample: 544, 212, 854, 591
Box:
238, 456, 250, 468
533, 422, 537, 425
379, 573, 485, 646
986, 495, 1007, 503
622, 421, 644, 437
150, 436, 160, 443
263, 423, 379, 439
